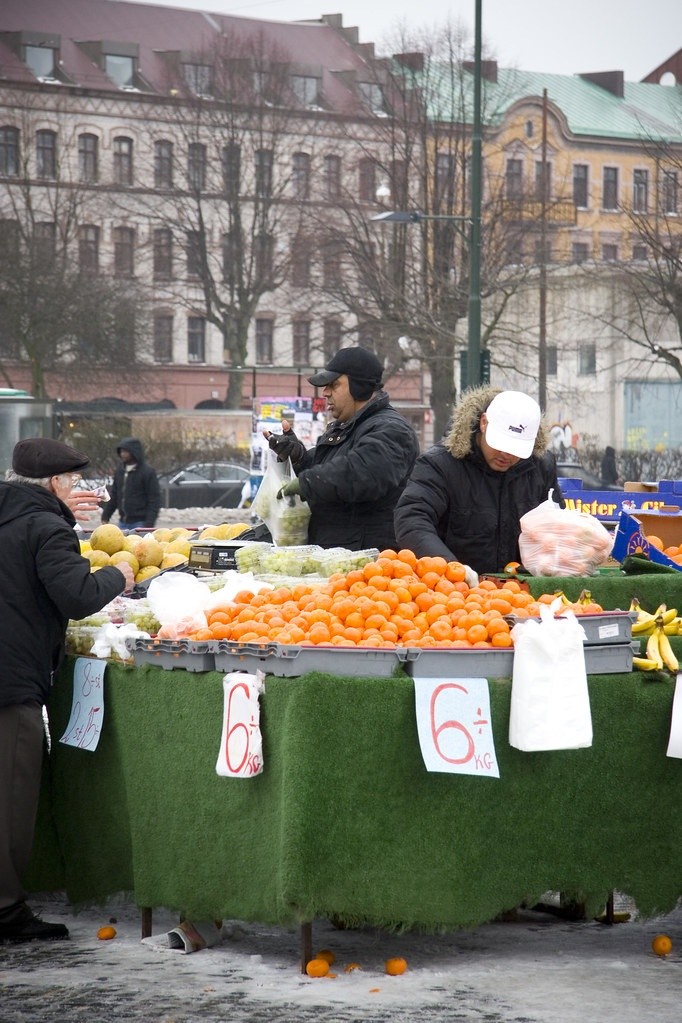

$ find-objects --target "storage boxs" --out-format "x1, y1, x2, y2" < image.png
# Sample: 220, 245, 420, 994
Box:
65, 474, 682, 675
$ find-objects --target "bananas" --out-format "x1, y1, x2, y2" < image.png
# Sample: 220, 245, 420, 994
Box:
594, 911, 631, 923
554, 590, 682, 673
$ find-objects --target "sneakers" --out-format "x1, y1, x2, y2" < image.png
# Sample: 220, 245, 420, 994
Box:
0, 905, 70, 944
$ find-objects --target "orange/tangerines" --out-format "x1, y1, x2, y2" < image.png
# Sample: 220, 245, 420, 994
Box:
385, 957, 407, 975
147, 547, 605, 648
97, 926, 116, 940
645, 534, 682, 567
316, 949, 336, 965
518, 516, 608, 577
306, 958, 329, 977
652, 935, 672, 955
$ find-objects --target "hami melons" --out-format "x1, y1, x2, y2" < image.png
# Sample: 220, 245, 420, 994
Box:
78, 524, 252, 582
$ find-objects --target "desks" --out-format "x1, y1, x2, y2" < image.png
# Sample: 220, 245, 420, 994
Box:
54, 679, 681, 972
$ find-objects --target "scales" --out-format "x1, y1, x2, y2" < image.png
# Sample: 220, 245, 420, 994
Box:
188, 540, 275, 571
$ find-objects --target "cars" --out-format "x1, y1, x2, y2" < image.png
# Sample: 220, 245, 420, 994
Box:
155, 461, 252, 509
558, 462, 626, 491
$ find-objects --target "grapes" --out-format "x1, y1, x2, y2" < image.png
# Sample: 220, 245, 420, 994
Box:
253, 480, 310, 545
64, 547, 377, 662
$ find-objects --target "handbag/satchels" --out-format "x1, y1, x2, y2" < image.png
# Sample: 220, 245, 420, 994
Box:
517, 487, 615, 579
249, 432, 312, 548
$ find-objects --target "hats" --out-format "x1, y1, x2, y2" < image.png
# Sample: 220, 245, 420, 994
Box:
486, 390, 541, 459
306, 347, 385, 402
12, 438, 90, 479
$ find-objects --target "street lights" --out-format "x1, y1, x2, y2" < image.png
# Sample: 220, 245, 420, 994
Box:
368, 212, 484, 390
237, 365, 256, 398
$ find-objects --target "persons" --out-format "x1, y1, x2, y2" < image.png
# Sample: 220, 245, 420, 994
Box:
261, 347, 421, 550
601, 445, 620, 485
0, 438, 134, 943
395, 383, 565, 589
101, 438, 162, 538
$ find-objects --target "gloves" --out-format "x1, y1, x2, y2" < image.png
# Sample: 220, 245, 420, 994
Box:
276, 478, 307, 503
462, 565, 479, 589
